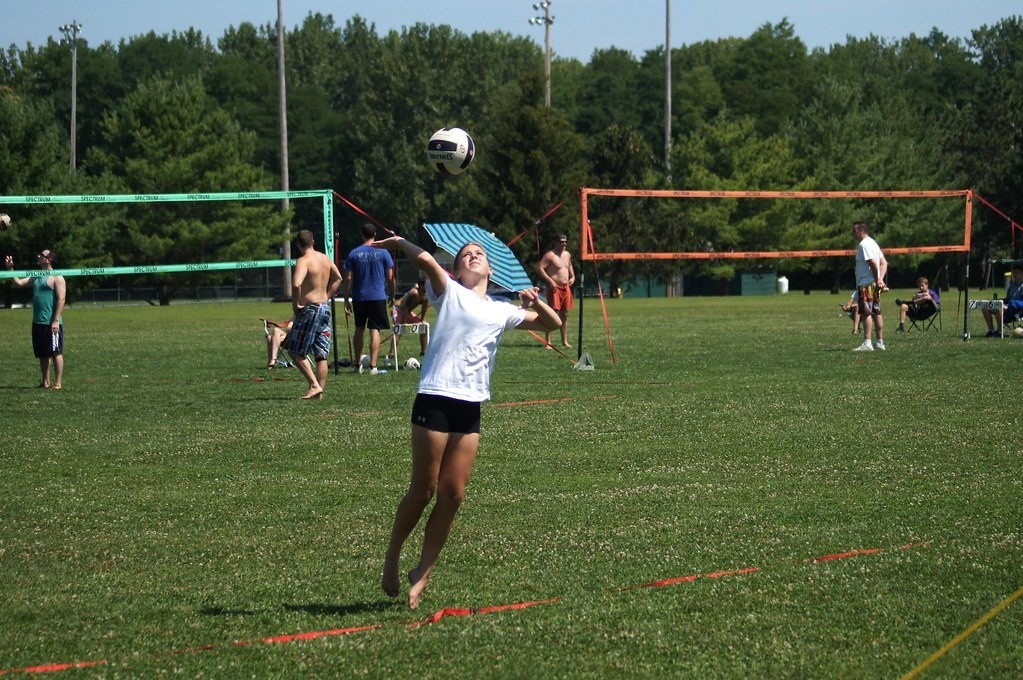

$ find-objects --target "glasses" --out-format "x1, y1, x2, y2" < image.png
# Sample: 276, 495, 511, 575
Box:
41, 254, 49, 259
556, 241, 567, 244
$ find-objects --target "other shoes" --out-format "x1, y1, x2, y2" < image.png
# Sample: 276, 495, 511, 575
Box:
843, 305, 853, 312
851, 329, 860, 334
895, 298, 903, 306
985, 329, 1004, 338
368, 364, 378, 376
895, 327, 904, 334
354, 363, 364, 374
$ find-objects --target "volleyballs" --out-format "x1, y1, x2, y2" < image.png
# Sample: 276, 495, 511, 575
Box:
428, 127, 475, 175
0, 214, 11, 231
403, 357, 420, 371
361, 355, 371, 364
1014, 327, 1023, 337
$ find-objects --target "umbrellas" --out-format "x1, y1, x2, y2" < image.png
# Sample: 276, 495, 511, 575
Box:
423, 224, 534, 294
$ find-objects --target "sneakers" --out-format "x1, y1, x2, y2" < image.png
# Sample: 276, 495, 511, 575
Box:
873, 343, 886, 350
851, 343, 874, 351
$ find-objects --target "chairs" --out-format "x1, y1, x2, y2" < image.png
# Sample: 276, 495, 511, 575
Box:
903, 285, 942, 335
260, 317, 316, 368
993, 298, 1023, 338
839, 303, 862, 332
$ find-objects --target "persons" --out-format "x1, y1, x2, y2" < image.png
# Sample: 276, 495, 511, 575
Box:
344, 224, 396, 375
842, 290, 861, 333
538, 233, 576, 349
371, 236, 562, 610
6, 248, 67, 390
265, 314, 294, 370
286, 231, 342, 400
388, 279, 430, 358
983, 263, 1023, 337
852, 222, 888, 351
894, 277, 941, 332
